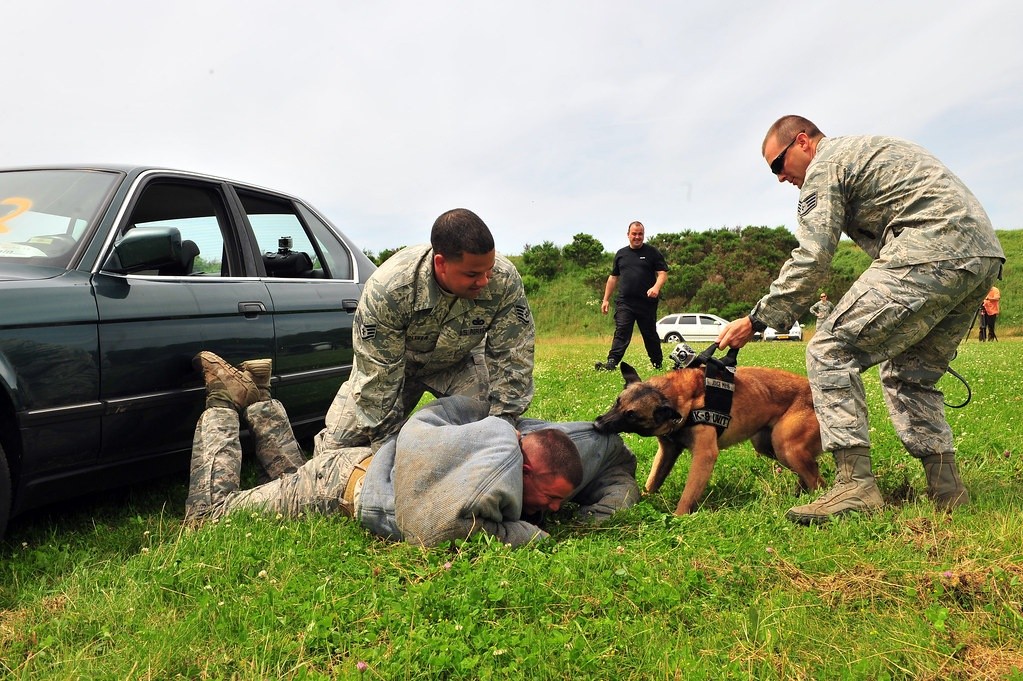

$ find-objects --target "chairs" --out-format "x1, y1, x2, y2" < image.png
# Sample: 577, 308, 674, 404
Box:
133, 240, 200, 276
262, 249, 314, 281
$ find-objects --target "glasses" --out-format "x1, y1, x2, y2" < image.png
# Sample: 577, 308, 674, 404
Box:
771, 130, 806, 174
821, 296, 826, 298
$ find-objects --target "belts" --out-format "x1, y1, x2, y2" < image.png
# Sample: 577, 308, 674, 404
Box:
339, 455, 375, 518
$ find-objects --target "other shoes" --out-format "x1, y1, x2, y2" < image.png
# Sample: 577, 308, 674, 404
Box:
595, 358, 615, 371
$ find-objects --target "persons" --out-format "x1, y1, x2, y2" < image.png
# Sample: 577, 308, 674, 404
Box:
313, 211, 536, 458
185, 351, 584, 549
594, 221, 668, 372
716, 115, 1007, 528
809, 292, 834, 332
979, 286, 1000, 342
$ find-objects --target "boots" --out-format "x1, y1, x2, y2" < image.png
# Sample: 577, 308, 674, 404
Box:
921, 453, 969, 512
786, 447, 885, 524
191, 351, 260, 409
236, 359, 273, 403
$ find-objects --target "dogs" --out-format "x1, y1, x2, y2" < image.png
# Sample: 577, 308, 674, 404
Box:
592, 366, 826, 516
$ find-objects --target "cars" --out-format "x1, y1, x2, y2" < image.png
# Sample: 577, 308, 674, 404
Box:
0, 165, 388, 535
764, 319, 807, 342
654, 313, 761, 344
